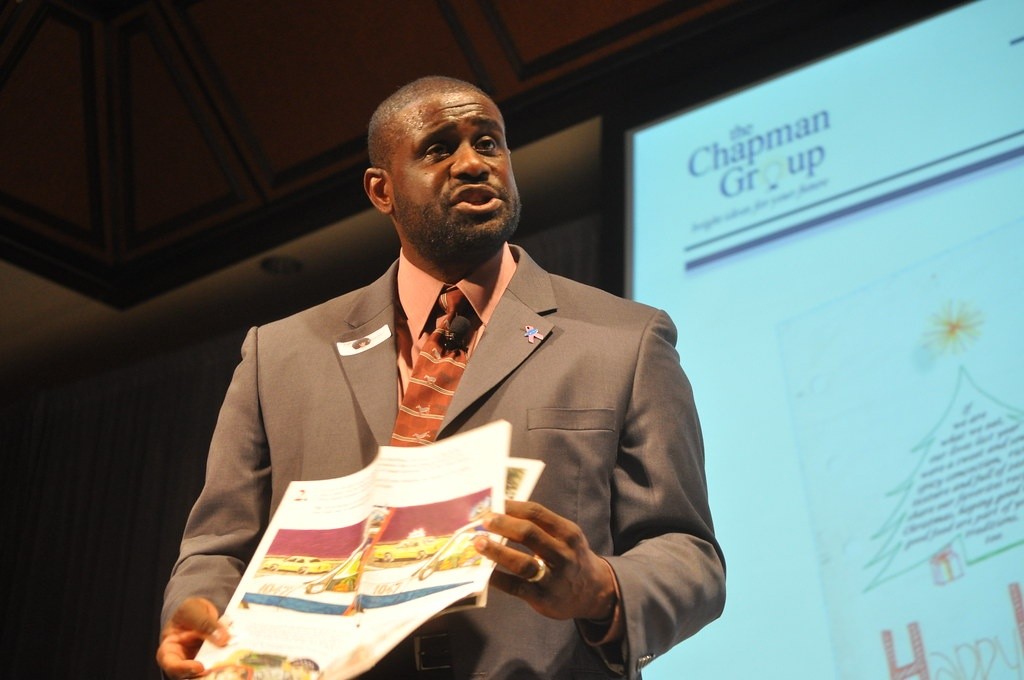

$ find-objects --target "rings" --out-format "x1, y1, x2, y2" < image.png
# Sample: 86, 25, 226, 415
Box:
527, 557, 546, 582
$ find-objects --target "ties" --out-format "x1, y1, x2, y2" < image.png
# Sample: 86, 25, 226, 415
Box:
389, 286, 473, 448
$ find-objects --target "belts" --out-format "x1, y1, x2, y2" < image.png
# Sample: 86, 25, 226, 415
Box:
368, 630, 453, 677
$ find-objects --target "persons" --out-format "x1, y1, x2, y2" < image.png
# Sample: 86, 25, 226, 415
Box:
154, 76, 726, 680
